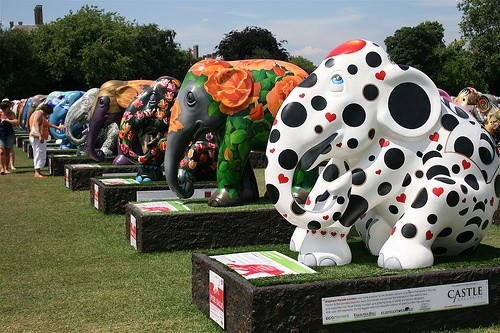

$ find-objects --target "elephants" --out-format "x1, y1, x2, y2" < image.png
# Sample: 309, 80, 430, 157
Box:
264, 39, 500, 271
13, 37, 318, 207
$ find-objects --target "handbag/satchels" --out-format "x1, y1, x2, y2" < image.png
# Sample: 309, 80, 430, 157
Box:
29, 123, 44, 137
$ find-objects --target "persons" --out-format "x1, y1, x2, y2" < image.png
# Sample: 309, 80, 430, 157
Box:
0, 100, 19, 175
30, 103, 66, 178
4, 101, 17, 171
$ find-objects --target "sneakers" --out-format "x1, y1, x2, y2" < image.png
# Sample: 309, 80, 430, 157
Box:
33, 173, 45, 178
0, 169, 4, 175
4, 170, 10, 173
